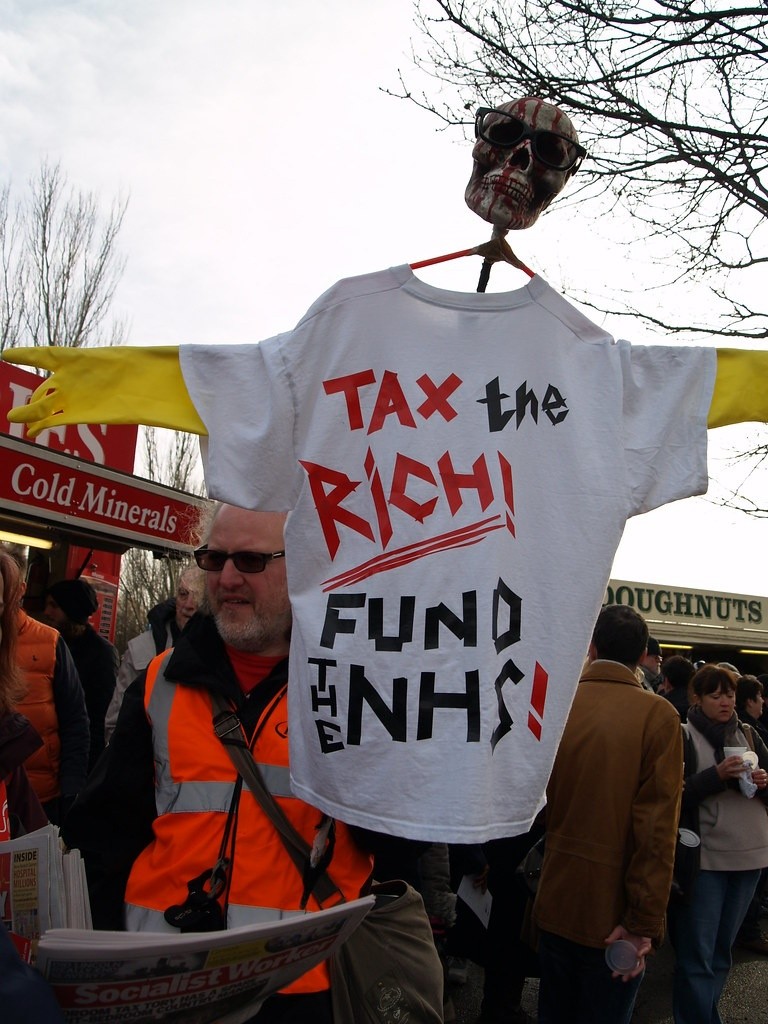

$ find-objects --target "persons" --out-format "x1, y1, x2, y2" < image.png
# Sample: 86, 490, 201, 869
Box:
525, 605, 768, 1024
0, 505, 540, 1023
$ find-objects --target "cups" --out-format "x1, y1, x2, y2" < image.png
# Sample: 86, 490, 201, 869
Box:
723, 746, 747, 775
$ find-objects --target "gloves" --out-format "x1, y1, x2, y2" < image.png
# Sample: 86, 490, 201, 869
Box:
427, 915, 449, 947
55, 794, 91, 827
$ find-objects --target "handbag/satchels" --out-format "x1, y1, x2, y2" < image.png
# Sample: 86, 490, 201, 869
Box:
673, 729, 702, 876
325, 879, 445, 1024
513, 836, 545, 892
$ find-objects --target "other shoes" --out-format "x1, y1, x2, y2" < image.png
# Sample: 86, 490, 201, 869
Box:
442, 950, 477, 989
480, 1000, 533, 1024
735, 928, 768, 955
759, 896, 768, 918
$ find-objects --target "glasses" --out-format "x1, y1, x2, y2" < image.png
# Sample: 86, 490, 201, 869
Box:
653, 655, 663, 664
474, 107, 588, 176
193, 544, 285, 573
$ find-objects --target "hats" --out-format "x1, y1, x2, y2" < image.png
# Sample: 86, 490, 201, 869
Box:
48, 579, 99, 625
647, 638, 662, 654
693, 659, 707, 670
715, 662, 743, 679
